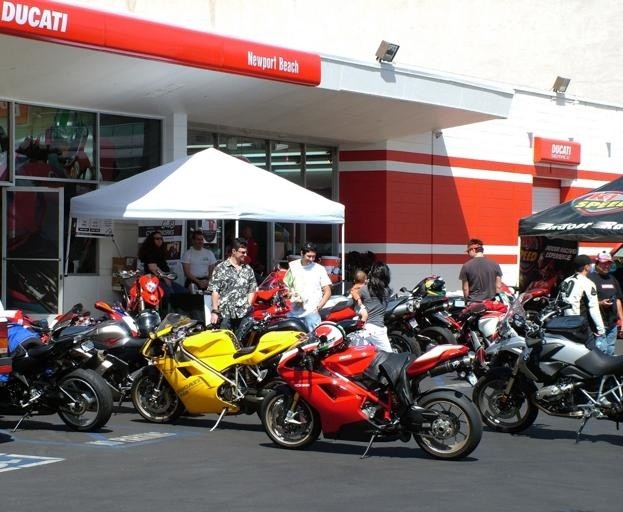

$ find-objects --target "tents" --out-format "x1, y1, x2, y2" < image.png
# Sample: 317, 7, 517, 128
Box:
515, 174, 623, 298
65, 147, 346, 296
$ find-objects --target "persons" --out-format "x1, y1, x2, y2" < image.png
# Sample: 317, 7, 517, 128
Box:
136, 231, 171, 318
283, 242, 334, 332
346, 270, 366, 299
180, 230, 217, 289
352, 261, 392, 354
459, 238, 503, 306
559, 254, 605, 350
207, 238, 258, 331
589, 250, 623, 357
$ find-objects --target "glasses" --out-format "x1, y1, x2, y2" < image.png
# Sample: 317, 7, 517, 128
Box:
237, 251, 248, 254
153, 238, 162, 240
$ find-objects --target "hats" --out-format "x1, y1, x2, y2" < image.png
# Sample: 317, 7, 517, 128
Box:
464, 244, 481, 252
595, 250, 614, 263
576, 255, 594, 265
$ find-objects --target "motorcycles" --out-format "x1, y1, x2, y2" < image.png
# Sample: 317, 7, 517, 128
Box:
131, 316, 311, 432
259, 322, 483, 462
469, 292, 623, 443
52, 302, 162, 416
111, 267, 166, 312
235, 312, 311, 348
385, 295, 423, 358
0, 321, 133, 433
249, 269, 366, 325
416, 295, 456, 355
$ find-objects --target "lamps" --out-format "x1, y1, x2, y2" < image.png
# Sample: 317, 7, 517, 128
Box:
554, 77, 570, 95
374, 40, 399, 65
